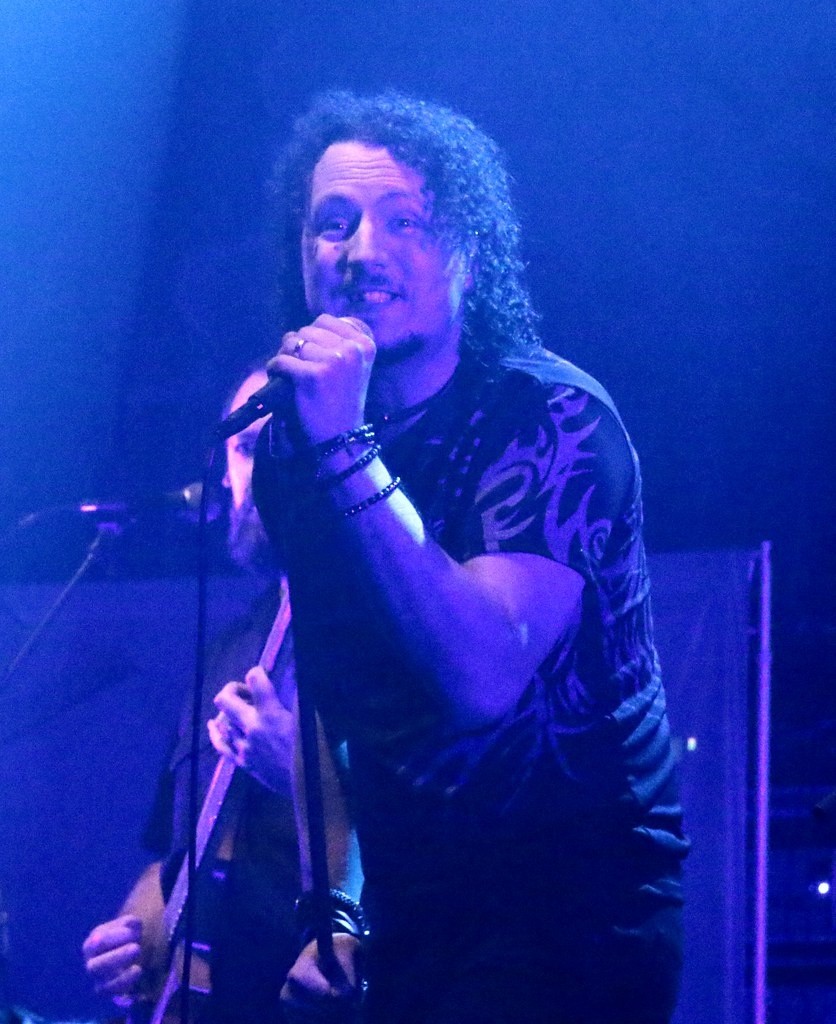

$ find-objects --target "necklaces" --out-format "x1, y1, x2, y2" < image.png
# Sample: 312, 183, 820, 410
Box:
368, 367, 456, 422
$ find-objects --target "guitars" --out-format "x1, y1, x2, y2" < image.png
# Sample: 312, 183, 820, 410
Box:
107, 571, 296, 1024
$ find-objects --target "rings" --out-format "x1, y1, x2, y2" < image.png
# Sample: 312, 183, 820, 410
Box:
292, 339, 305, 358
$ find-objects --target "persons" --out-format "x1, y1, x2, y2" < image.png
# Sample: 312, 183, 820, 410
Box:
83, 358, 349, 1024
266, 93, 693, 1024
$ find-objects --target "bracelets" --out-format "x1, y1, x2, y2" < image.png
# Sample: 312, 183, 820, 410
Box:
310, 424, 401, 526
289, 887, 374, 949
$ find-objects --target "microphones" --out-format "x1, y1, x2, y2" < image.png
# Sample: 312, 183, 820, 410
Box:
24, 484, 221, 532
215, 317, 374, 435
